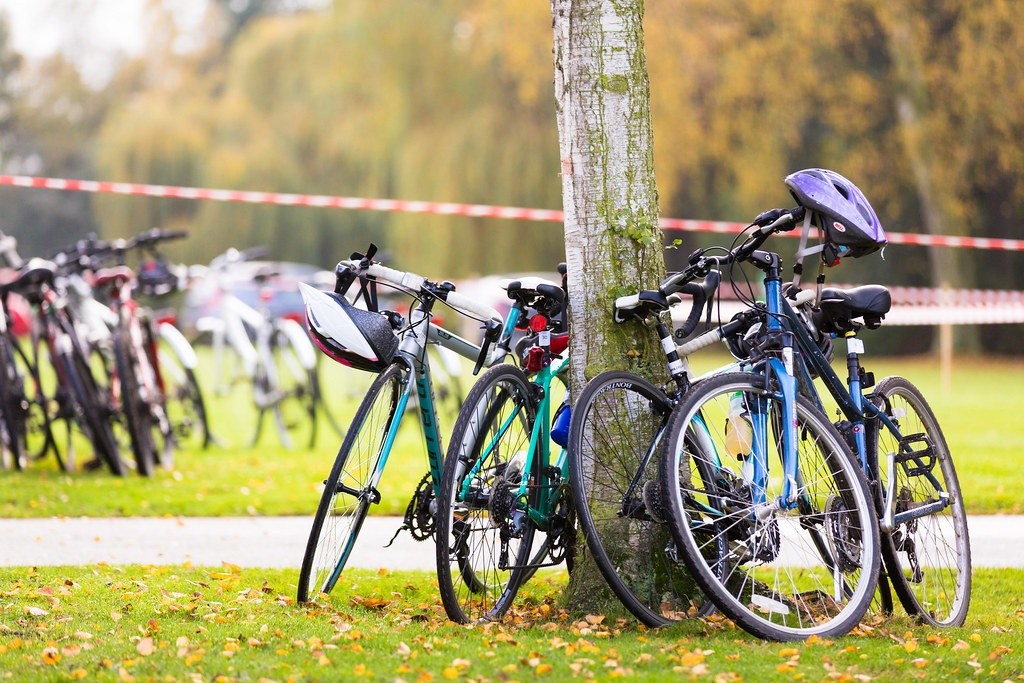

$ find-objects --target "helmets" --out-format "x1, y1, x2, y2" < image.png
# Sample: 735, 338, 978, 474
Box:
297, 279, 405, 374
783, 166, 888, 268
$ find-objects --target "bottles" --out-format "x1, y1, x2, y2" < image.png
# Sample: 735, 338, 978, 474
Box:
548, 385, 576, 449
725, 390, 757, 457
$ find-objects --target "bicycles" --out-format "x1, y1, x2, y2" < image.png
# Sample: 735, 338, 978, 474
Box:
0, 229, 322, 478
295, 199, 973, 643
